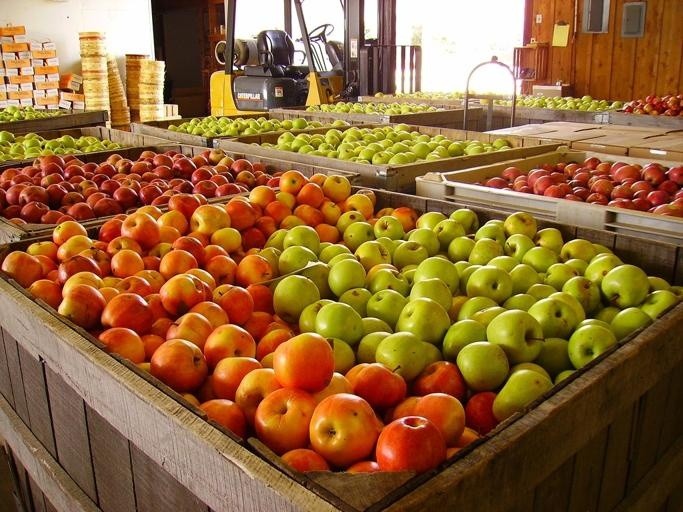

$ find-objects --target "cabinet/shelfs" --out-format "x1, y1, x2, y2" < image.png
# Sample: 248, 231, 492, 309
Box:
513, 46, 548, 82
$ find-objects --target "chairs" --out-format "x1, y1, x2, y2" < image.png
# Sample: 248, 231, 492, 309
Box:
259, 30, 309, 78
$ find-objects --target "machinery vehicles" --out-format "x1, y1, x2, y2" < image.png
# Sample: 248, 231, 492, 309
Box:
210, 0, 421, 118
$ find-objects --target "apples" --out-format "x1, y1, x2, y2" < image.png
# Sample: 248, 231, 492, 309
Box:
1, 104, 68, 124
250, 122, 512, 166
1, 131, 121, 160
375, 91, 466, 101
0, 170, 682, 478
306, 102, 445, 115
479, 94, 683, 117
1, 147, 285, 225
475, 156, 682, 219
168, 116, 350, 137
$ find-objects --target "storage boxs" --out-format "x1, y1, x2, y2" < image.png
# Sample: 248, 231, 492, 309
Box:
0, 94, 683, 512
0, 25, 83, 99
